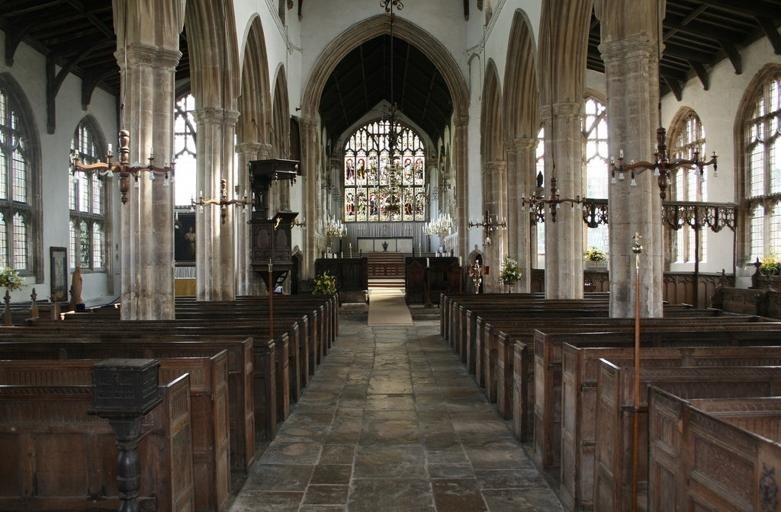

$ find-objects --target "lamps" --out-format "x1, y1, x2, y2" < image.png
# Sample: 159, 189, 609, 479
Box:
191, 0, 258, 226
67, 0, 178, 206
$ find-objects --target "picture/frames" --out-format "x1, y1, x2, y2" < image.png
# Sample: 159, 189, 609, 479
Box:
173, 211, 196, 268
48, 246, 69, 302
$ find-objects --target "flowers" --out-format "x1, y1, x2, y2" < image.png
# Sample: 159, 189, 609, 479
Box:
0, 264, 29, 293
308, 270, 339, 297
760, 253, 779, 277
587, 245, 608, 265
499, 253, 524, 287
